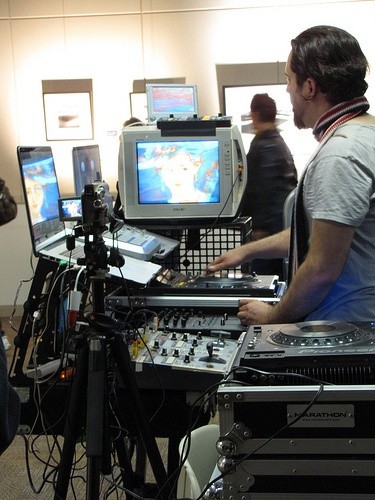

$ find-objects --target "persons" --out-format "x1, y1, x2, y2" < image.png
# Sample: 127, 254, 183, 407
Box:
206, 25, 375, 326
235, 92, 298, 281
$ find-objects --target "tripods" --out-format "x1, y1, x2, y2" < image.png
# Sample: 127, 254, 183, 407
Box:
52, 225, 174, 499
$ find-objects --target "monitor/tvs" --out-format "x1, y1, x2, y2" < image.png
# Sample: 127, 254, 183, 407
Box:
145, 83, 198, 123
122, 126, 248, 219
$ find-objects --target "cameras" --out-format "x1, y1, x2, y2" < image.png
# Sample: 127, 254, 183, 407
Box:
58, 180, 114, 232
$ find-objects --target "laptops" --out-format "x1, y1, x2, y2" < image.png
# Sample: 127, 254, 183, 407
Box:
72, 144, 181, 259
16, 146, 162, 285
